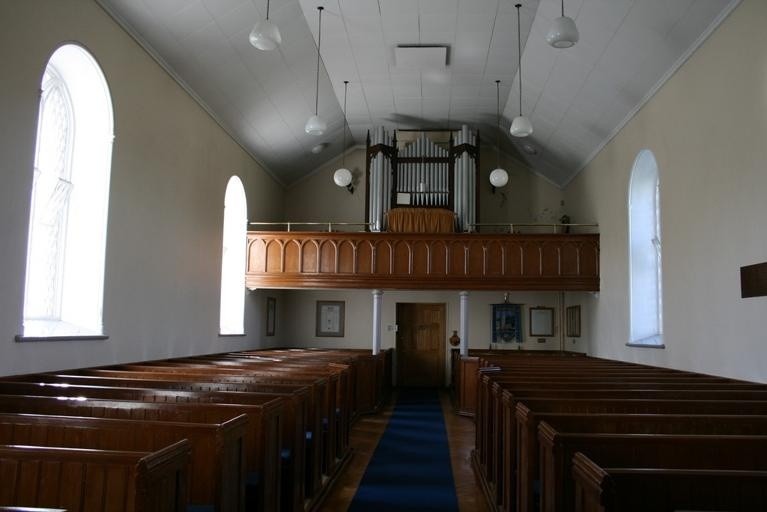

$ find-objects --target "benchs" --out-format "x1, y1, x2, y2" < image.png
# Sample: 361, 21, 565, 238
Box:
451, 347, 767, 512
0, 343, 396, 512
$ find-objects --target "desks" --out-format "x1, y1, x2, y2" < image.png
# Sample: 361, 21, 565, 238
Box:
383, 204, 454, 234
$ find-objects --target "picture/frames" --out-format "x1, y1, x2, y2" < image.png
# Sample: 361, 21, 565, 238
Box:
530, 306, 555, 337
315, 299, 345, 338
566, 304, 581, 338
265, 296, 276, 336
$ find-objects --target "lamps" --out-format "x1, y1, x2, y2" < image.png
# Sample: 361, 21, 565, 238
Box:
333, 80, 353, 187
488, 75, 509, 188
507, 0, 534, 137
305, 4, 330, 136
544, 1, 579, 48
248, 0, 282, 50
